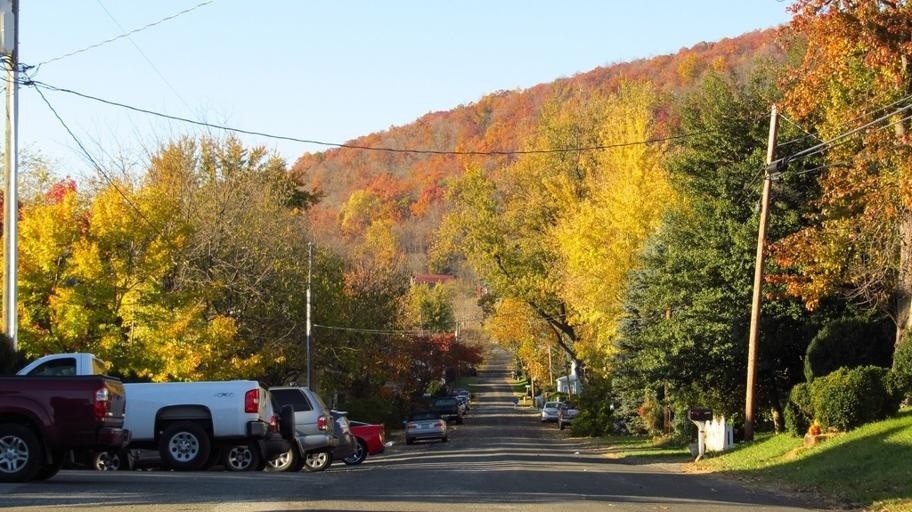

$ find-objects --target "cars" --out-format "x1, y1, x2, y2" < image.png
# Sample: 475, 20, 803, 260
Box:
433, 390, 471, 424
265, 385, 387, 473
541, 401, 579, 430
404, 411, 448, 445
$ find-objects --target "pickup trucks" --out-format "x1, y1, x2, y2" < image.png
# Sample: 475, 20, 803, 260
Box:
1, 332, 126, 485
17, 352, 278, 471
